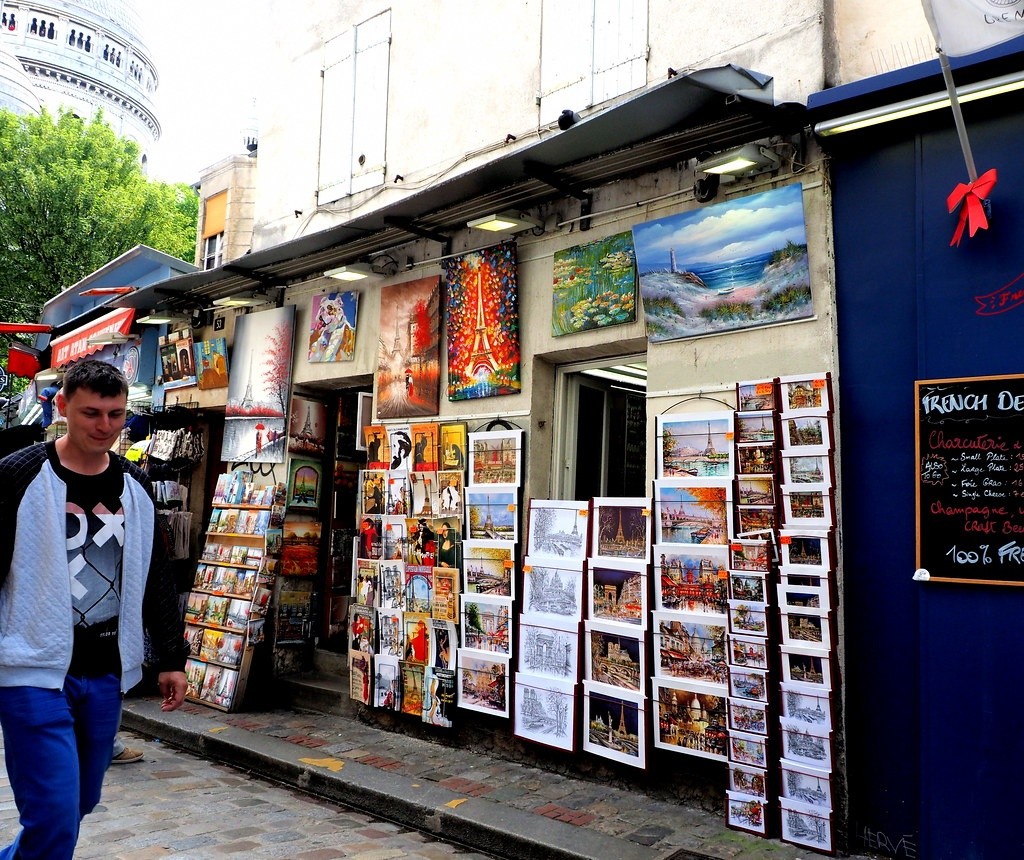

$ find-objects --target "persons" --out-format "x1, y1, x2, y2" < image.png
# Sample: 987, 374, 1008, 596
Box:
0, 360, 189, 860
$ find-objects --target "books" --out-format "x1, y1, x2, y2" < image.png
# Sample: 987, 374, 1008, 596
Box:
180, 471, 286, 710
348, 421, 463, 729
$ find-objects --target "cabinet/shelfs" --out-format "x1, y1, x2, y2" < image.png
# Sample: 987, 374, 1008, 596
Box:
171, 503, 279, 713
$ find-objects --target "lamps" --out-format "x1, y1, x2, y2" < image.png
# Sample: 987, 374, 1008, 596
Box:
467, 209, 544, 235
34, 368, 65, 380
86, 332, 140, 345
135, 310, 192, 325
323, 262, 386, 281
694, 144, 783, 179
213, 291, 277, 307
